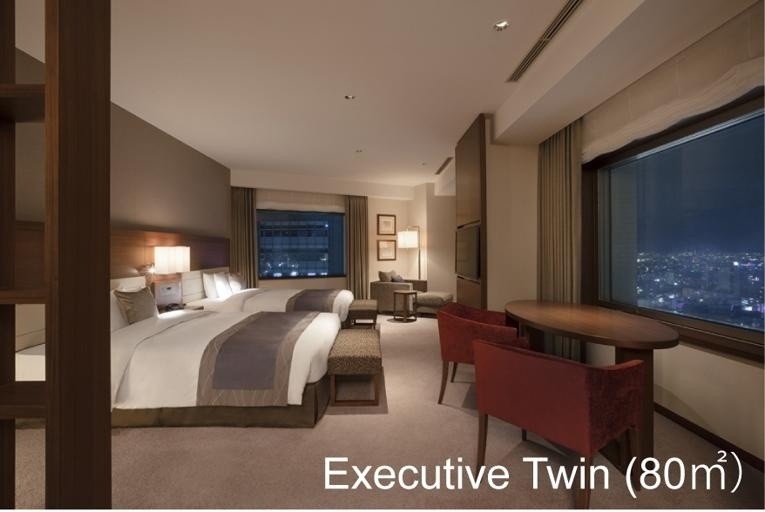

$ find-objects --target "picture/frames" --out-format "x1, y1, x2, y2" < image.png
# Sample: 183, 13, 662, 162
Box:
377, 214, 396, 235
377, 240, 396, 261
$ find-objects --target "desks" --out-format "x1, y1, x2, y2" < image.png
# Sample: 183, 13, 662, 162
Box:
505, 300, 679, 476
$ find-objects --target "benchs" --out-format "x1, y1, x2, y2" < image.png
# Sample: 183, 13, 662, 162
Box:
349, 299, 378, 327
328, 328, 382, 406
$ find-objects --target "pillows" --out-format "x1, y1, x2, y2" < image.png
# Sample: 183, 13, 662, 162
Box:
378, 270, 397, 282
391, 275, 405, 282
213, 272, 233, 298
203, 272, 213, 298
226, 272, 247, 292
113, 286, 160, 325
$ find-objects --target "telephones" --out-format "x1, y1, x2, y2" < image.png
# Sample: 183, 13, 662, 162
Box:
165, 302, 185, 310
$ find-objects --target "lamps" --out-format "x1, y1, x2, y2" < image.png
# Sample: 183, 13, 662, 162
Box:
398, 226, 420, 280
144, 245, 190, 274
14, 222, 341, 429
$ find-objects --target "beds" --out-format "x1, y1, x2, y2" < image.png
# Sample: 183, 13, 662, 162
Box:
182, 266, 355, 328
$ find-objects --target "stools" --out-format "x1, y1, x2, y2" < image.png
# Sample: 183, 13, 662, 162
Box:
415, 291, 453, 317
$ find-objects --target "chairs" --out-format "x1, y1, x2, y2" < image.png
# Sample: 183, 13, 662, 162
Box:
472, 337, 645, 509
437, 301, 518, 405
370, 280, 428, 317
